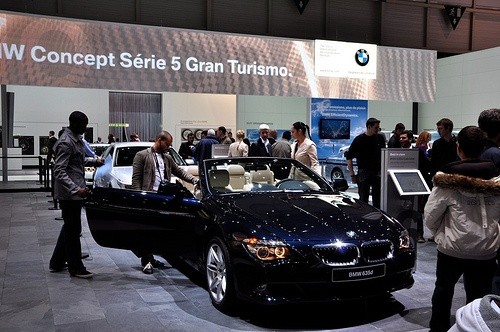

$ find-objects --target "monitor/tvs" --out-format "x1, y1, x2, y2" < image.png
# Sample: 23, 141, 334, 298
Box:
319, 118, 351, 140
386, 169, 432, 196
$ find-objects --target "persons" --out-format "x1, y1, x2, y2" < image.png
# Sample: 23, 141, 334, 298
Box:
346, 118, 386, 209
416, 132, 431, 162
431, 119, 458, 176
130, 134, 140, 142
108, 134, 115, 144
132, 131, 200, 274
49, 111, 104, 278
478, 109, 500, 294
37, 131, 57, 175
271, 131, 292, 158
423, 126, 500, 332
400, 130, 413, 148
58, 129, 97, 157
196, 124, 277, 168
388, 123, 405, 148
94, 138, 103, 143
447, 294, 500, 332
178, 133, 196, 160
288, 122, 318, 180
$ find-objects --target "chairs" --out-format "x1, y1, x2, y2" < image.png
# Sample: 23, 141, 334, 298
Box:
249, 164, 273, 190
210, 169, 231, 194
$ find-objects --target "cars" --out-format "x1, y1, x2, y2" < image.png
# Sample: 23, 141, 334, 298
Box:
91, 142, 188, 189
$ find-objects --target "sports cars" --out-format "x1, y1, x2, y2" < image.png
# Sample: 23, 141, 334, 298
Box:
84, 156, 418, 311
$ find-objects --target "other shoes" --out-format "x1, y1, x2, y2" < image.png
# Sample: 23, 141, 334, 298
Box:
418, 236, 424, 242
142, 260, 153, 273
49, 262, 69, 271
141, 257, 164, 267
69, 266, 94, 278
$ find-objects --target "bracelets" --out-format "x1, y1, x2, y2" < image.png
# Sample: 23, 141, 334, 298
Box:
351, 175, 355, 178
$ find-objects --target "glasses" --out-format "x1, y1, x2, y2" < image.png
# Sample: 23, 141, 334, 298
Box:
164, 139, 172, 148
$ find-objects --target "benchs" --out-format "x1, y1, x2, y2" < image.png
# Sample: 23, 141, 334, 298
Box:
181, 164, 249, 192
